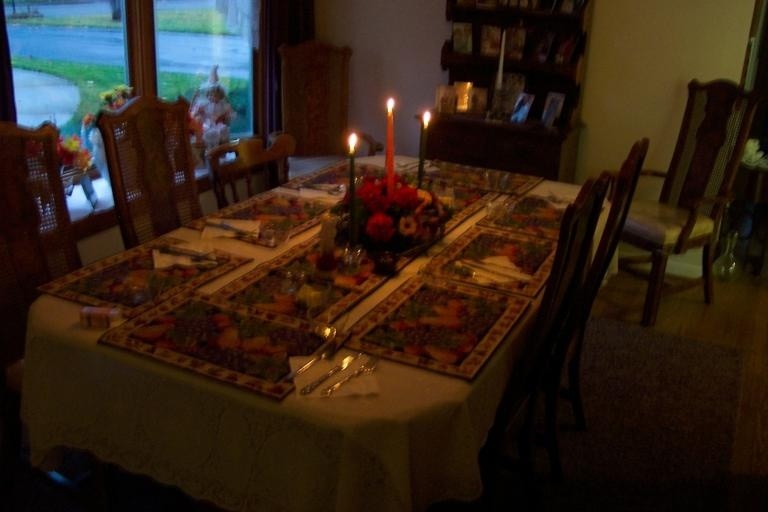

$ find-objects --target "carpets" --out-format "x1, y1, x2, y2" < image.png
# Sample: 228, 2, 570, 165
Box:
471, 324, 742, 512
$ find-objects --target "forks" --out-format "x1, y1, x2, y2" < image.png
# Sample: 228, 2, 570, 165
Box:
321, 353, 380, 398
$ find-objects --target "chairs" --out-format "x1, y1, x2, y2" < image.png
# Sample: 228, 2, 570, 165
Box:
616, 78, 759, 327
498, 140, 646, 470
93, 94, 204, 247
208, 132, 295, 204
5, 119, 80, 294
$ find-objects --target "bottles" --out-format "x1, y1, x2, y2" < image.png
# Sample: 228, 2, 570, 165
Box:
434, 85, 456, 116
80, 307, 122, 330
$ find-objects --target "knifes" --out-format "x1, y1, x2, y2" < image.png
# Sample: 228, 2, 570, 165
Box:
299, 350, 364, 396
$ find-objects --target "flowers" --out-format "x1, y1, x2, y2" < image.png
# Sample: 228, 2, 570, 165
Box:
344, 177, 447, 264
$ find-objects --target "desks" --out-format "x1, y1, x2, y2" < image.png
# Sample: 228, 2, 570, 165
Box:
3, 147, 383, 454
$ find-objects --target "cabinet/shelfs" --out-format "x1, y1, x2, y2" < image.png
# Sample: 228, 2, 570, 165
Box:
440, 0, 593, 134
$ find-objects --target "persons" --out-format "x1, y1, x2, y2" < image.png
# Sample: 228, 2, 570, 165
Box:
191, 86, 234, 145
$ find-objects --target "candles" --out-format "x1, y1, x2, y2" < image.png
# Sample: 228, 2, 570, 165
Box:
417, 123, 428, 183
387, 109, 395, 177
